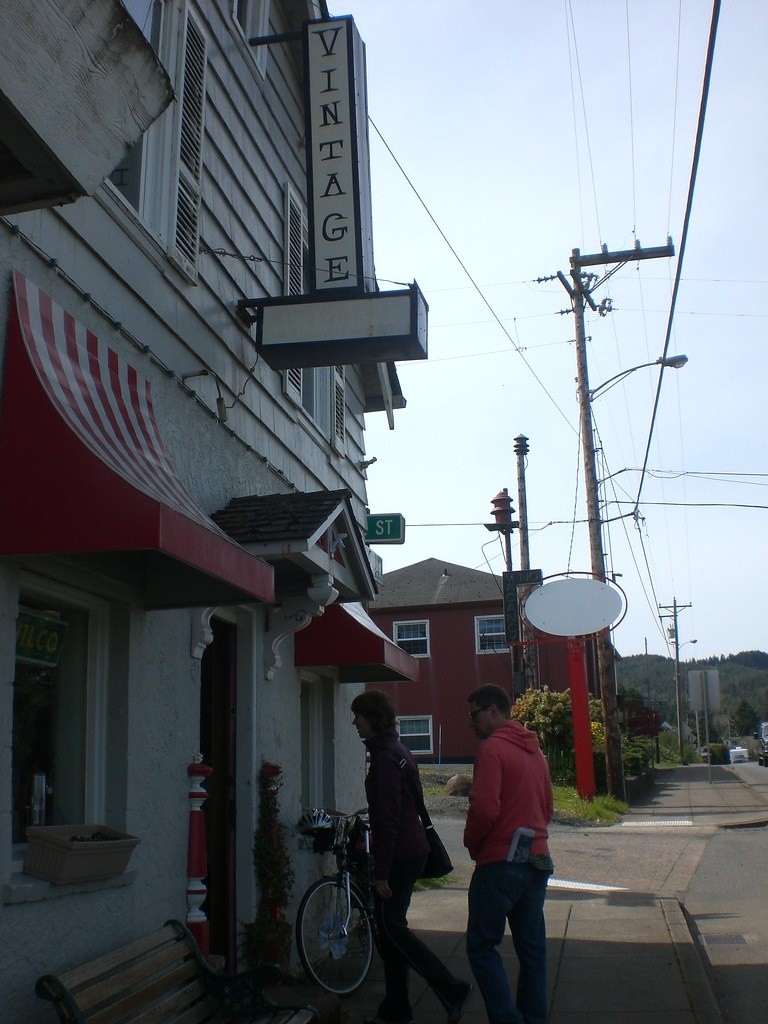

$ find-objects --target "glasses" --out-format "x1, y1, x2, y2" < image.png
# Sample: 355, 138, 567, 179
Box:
468, 703, 491, 721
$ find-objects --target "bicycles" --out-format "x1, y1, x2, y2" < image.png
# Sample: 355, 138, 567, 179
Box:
296, 806, 385, 997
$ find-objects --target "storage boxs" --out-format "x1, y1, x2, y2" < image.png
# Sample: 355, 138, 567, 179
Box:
23, 823, 140, 884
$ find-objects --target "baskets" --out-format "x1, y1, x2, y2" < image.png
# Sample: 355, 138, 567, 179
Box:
304, 814, 346, 851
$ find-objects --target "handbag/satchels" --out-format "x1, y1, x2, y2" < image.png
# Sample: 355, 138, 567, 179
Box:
420, 827, 453, 879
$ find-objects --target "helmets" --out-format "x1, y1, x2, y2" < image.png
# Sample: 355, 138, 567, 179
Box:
297, 808, 333, 833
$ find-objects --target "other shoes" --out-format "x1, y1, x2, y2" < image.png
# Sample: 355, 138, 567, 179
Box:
448, 982, 472, 1024
369, 1014, 415, 1024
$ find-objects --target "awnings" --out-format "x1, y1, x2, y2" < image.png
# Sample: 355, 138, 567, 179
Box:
295, 600, 419, 685
1, 266, 278, 610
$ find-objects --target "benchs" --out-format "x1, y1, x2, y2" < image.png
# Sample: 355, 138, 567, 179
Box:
36, 919, 324, 1024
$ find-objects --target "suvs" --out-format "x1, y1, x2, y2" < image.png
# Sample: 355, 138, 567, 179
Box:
752, 721, 768, 767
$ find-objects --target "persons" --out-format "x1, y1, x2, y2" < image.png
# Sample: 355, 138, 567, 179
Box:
444, 684, 553, 1023
349, 687, 474, 1023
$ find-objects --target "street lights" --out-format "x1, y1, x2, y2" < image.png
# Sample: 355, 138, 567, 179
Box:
674, 638, 698, 765
582, 354, 687, 807
